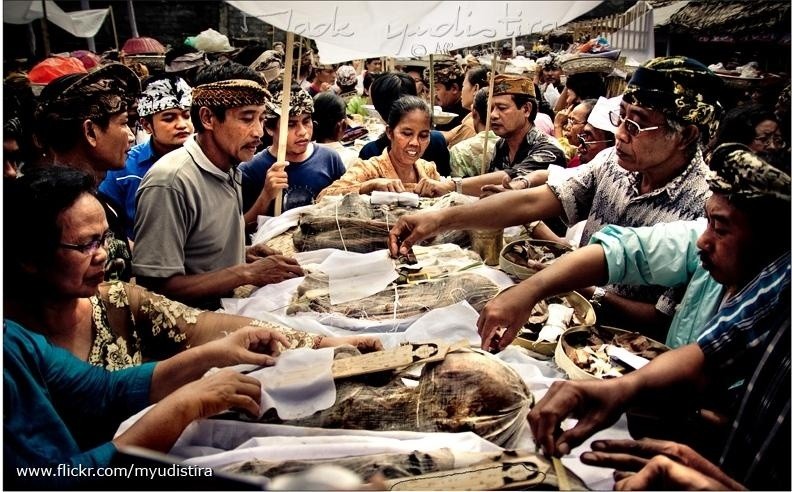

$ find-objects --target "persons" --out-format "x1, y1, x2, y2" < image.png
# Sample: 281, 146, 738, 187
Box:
3, 30, 792, 492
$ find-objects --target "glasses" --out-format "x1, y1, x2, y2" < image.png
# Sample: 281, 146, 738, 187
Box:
59, 228, 115, 257
609, 109, 666, 138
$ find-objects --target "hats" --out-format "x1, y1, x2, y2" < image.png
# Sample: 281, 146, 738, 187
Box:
335, 65, 357, 87
486, 72, 536, 97
622, 54, 726, 146
